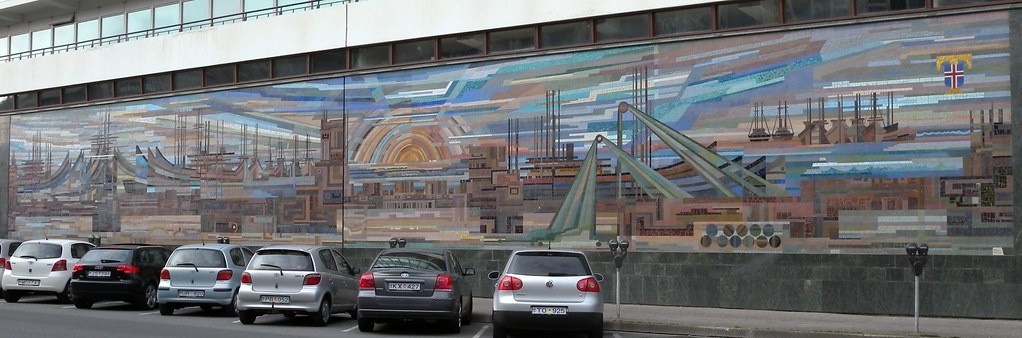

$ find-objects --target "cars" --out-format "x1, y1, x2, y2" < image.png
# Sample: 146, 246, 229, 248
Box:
157, 244, 254, 315
356, 249, 476, 333
237, 244, 360, 327
488, 249, 604, 338
70, 243, 173, 311
0, 239, 95, 304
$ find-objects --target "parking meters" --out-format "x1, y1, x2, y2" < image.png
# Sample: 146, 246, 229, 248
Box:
608, 239, 630, 318
904, 243, 929, 332
217, 237, 229, 244
88, 235, 102, 246
387, 236, 406, 248
